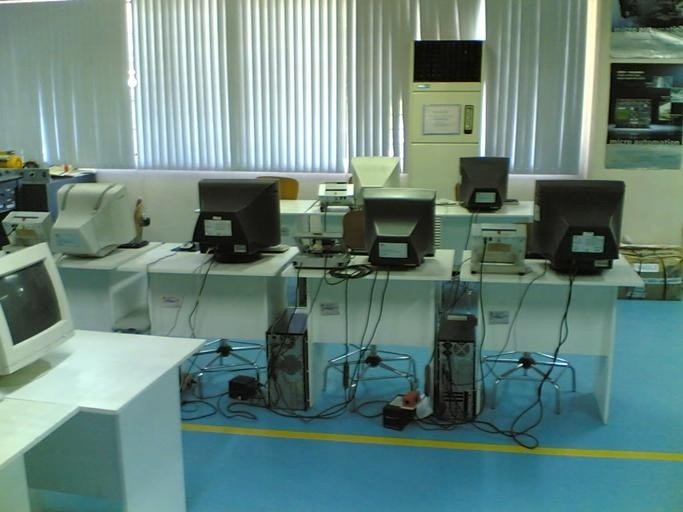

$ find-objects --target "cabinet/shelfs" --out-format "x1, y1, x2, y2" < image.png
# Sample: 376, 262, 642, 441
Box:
578, 0, 681, 250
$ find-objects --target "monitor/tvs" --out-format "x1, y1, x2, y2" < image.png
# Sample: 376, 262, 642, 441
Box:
48, 182, 137, 258
1, 240, 77, 377
360, 185, 439, 271
531, 177, 626, 278
191, 178, 283, 265
457, 156, 512, 215
349, 156, 401, 203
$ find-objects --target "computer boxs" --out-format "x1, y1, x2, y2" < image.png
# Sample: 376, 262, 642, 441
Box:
263, 307, 310, 412
432, 312, 477, 424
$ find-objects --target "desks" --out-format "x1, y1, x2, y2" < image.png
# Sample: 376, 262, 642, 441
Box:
50, 241, 165, 333
0, 397, 94, 508
0, 329, 206, 508
119, 243, 301, 410
280, 249, 455, 415
435, 200, 534, 269
193, 200, 317, 249
458, 249, 645, 426
